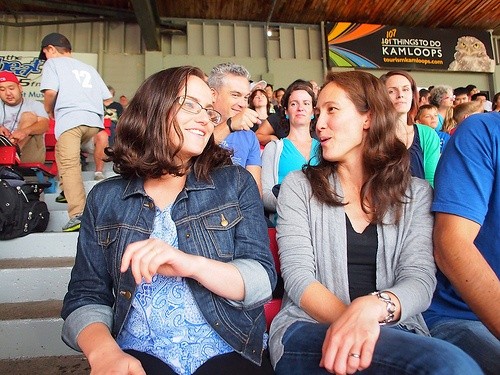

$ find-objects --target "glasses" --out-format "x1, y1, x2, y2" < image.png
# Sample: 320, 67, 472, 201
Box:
443, 94, 456, 101
175, 95, 222, 125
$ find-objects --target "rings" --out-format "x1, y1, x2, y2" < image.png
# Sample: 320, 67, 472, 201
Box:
348, 353, 360, 358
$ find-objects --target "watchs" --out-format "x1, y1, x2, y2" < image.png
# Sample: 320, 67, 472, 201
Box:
365, 291, 396, 325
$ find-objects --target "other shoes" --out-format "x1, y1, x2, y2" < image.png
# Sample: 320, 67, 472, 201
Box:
94, 172, 103, 180
62, 214, 82, 231
55, 191, 68, 203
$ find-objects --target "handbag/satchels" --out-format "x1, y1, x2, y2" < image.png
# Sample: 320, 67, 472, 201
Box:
0, 180, 50, 241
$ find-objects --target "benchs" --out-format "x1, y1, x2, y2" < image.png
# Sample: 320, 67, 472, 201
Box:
9, 115, 112, 173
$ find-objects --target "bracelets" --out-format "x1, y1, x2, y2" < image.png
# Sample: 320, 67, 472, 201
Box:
227, 117, 234, 132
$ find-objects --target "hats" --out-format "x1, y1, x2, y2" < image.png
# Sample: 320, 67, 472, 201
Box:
0, 71, 18, 84
250, 80, 266, 89
38, 32, 71, 60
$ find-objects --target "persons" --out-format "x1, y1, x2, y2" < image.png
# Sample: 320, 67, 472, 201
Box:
0, 62, 500, 375
39, 33, 111, 231
